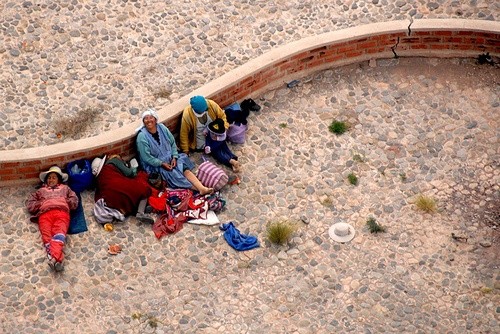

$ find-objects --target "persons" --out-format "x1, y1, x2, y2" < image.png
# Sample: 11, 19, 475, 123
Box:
181, 96, 240, 173
137, 110, 215, 196
26, 167, 79, 270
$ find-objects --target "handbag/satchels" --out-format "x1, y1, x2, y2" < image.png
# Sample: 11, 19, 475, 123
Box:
65, 159, 94, 192
206, 103, 248, 143
93, 198, 125, 223
194, 156, 228, 191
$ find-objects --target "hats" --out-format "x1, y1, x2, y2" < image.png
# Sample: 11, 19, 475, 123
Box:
329, 222, 356, 243
190, 95, 207, 114
90, 155, 107, 176
39, 166, 68, 182
141, 109, 159, 121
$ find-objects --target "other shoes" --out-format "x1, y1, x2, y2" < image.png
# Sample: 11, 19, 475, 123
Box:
135, 213, 154, 225
48, 260, 64, 271
